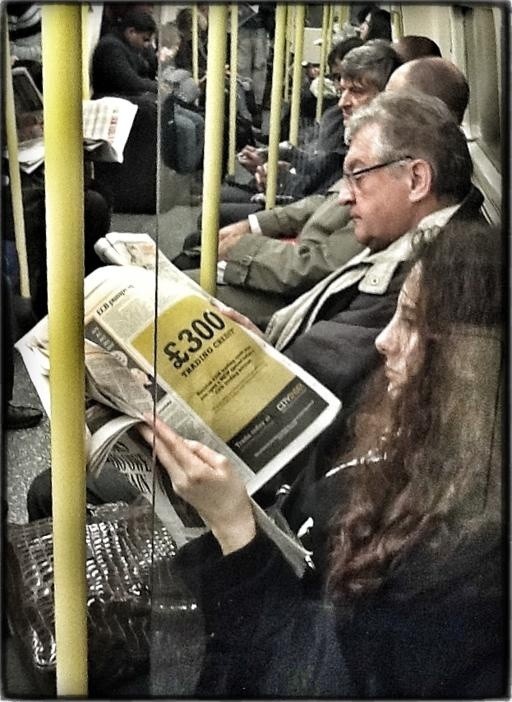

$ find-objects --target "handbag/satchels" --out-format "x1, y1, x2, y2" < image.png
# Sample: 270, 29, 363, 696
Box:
5, 498, 215, 697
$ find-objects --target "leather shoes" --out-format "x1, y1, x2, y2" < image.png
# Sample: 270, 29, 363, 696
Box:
8, 401, 44, 429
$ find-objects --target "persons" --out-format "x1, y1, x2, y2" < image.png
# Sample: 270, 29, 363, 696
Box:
5, 219, 506, 695
3, 2, 489, 511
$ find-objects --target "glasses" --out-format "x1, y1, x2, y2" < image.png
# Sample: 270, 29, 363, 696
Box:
341, 154, 414, 193
